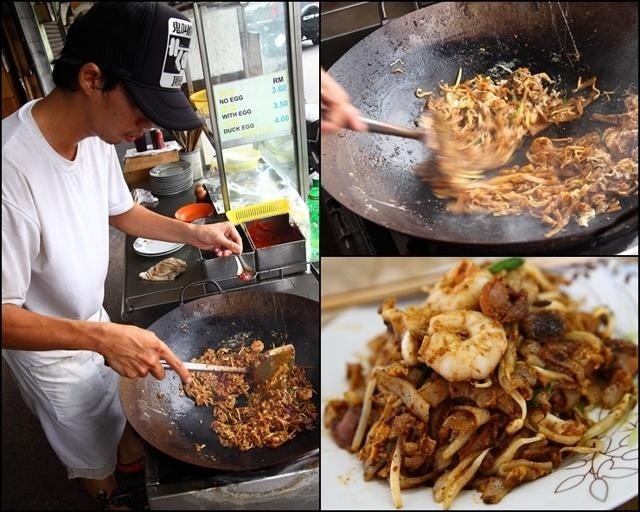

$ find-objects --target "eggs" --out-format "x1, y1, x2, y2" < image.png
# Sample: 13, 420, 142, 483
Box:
197, 190, 207, 201
195, 186, 204, 195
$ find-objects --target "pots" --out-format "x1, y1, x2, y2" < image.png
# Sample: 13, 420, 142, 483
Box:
321, 1, 639, 256
115, 278, 321, 476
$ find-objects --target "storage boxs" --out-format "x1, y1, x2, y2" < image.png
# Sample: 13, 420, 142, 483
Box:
122, 140, 180, 185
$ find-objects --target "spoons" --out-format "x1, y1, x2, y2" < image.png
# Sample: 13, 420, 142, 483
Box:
235, 249, 258, 283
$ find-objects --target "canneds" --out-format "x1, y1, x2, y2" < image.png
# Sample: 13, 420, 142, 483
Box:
149, 128, 164, 149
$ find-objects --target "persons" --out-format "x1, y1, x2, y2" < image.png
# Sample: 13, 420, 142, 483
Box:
1, 1, 250, 509
319, 70, 370, 135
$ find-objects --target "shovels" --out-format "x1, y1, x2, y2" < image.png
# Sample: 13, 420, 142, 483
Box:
132, 345, 297, 388
363, 112, 454, 153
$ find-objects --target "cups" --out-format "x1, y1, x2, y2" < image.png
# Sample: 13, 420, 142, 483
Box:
178, 146, 203, 182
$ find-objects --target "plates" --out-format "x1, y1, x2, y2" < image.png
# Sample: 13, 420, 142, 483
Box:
174, 203, 214, 222
148, 159, 194, 197
133, 236, 186, 257
321, 256, 639, 510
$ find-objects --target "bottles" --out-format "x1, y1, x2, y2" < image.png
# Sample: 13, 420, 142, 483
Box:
307, 176, 320, 250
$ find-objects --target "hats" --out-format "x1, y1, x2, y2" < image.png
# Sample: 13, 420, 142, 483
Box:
60, 1, 203, 131
